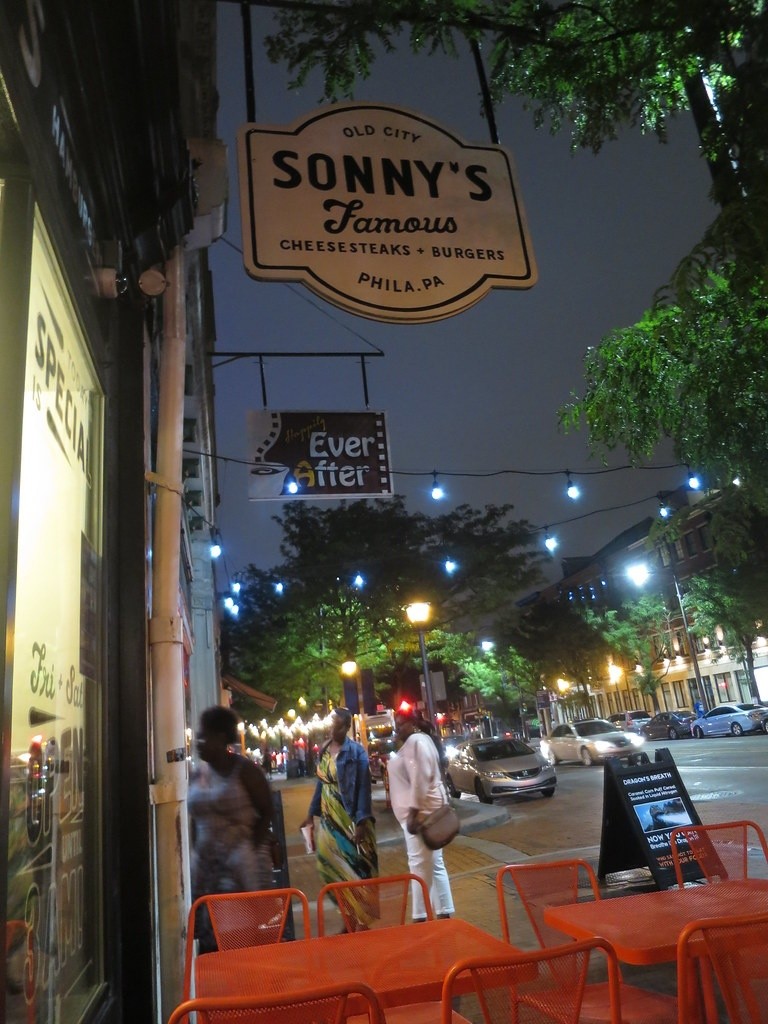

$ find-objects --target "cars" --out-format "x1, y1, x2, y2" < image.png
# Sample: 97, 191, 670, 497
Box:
369, 737, 404, 784
540, 719, 645, 765
691, 702, 768, 739
441, 735, 557, 804
638, 711, 699, 740
604, 710, 651, 736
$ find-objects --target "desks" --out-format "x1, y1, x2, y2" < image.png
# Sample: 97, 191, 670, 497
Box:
195, 919, 538, 1024
543, 878, 768, 1023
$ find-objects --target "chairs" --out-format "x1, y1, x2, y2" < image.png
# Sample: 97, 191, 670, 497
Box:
5, 920, 37, 1024
165, 873, 624, 1024
497, 820, 768, 1024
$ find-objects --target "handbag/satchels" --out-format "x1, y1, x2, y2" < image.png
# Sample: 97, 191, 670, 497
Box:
420, 805, 459, 850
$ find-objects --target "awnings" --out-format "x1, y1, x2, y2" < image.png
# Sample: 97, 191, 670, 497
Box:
225, 674, 276, 712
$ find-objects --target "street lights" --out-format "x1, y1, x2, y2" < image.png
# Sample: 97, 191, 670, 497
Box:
630, 567, 709, 713
608, 665, 624, 712
341, 659, 357, 741
403, 598, 437, 736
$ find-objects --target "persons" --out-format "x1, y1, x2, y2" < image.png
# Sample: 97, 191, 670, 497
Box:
624, 710, 629, 722
385, 707, 455, 922
186, 707, 278, 953
551, 719, 556, 728
694, 697, 704, 718
262, 749, 273, 781
300, 707, 379, 933
